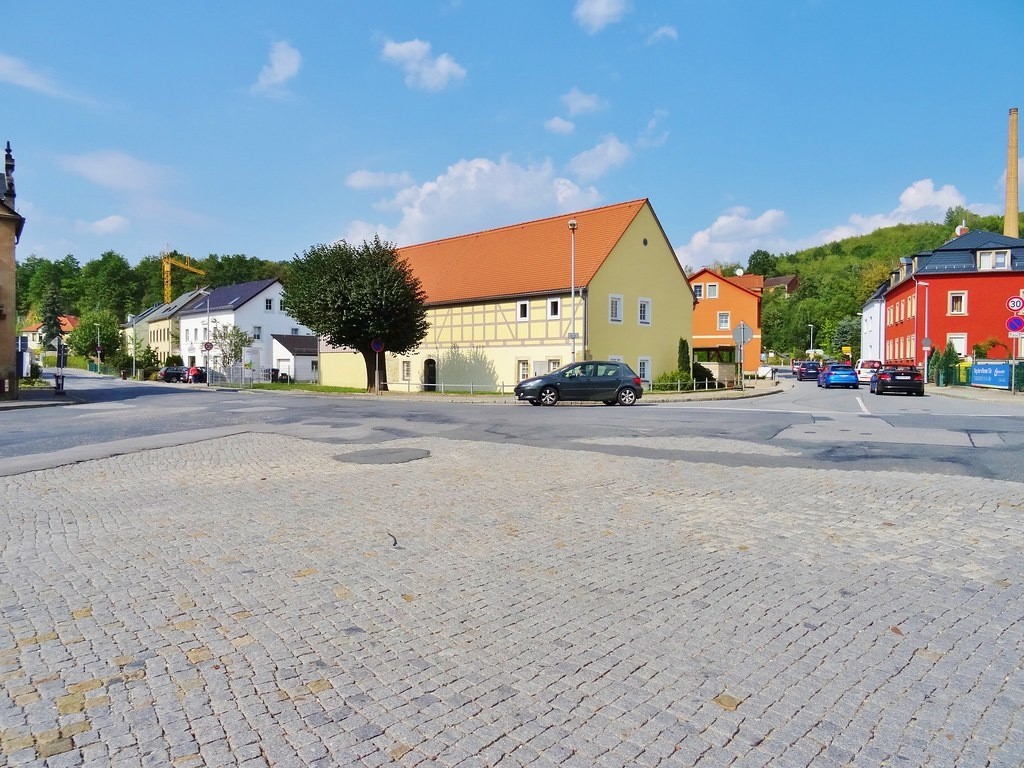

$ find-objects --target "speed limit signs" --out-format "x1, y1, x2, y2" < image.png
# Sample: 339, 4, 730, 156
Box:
1005, 295, 1024, 313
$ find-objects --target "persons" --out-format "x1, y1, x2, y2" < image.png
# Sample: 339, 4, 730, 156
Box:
187, 364, 198, 384
579, 367, 586, 377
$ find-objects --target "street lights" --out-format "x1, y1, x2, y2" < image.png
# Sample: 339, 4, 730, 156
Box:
568, 220, 578, 362
917, 281, 930, 383
200, 290, 210, 386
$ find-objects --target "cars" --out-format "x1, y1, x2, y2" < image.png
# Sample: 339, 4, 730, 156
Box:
514, 361, 644, 406
797, 361, 821, 381
179, 367, 226, 383
854, 358, 882, 385
817, 365, 859, 389
870, 363, 925, 396
157, 366, 190, 383
793, 360, 803, 375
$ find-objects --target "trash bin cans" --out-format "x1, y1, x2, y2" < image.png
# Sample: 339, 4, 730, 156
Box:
54, 374, 64, 389
